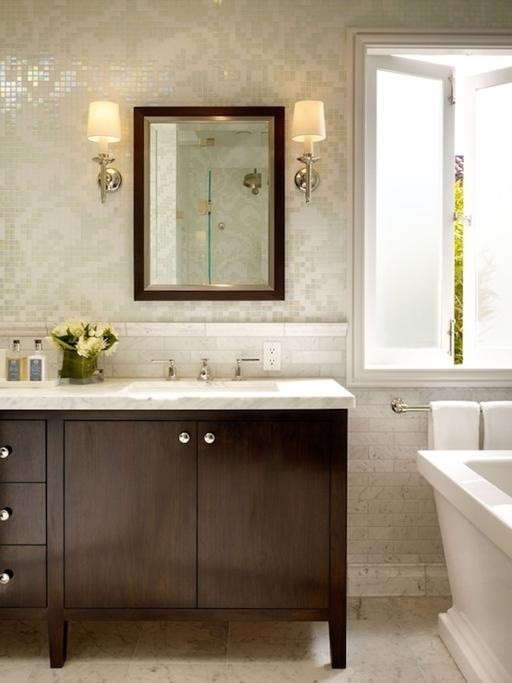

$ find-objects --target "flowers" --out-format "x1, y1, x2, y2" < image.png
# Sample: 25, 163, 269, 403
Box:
50, 318, 120, 359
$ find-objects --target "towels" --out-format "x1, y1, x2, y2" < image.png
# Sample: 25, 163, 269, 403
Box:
427, 401, 512, 450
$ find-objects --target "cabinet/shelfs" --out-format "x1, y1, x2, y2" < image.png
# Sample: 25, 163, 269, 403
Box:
0, 414, 49, 668
62, 411, 346, 671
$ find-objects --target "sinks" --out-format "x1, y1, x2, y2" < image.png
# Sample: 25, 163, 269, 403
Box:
116, 381, 281, 395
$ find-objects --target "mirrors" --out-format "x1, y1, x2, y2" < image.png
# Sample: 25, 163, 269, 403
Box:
132, 106, 285, 302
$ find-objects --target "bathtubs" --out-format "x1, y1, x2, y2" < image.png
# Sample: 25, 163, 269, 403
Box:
416, 449, 512, 560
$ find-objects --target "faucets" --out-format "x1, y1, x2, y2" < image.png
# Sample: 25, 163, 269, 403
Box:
198, 358, 212, 380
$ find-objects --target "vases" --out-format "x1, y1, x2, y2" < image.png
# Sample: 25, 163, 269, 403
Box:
60, 350, 97, 385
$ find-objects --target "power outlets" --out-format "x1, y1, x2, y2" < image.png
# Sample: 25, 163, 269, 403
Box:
263, 342, 281, 371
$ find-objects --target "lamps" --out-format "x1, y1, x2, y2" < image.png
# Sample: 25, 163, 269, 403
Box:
290, 100, 327, 206
87, 101, 121, 205
